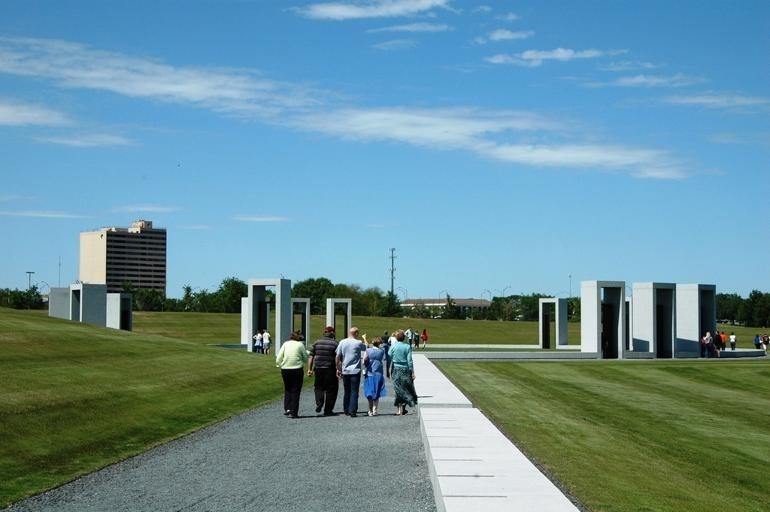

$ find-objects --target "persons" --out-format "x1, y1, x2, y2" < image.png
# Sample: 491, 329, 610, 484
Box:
307, 326, 343, 416
754, 333, 770, 354
275, 329, 309, 417
421, 328, 429, 349
362, 336, 385, 417
262, 329, 271, 354
729, 331, 736, 350
334, 326, 368, 417
701, 330, 727, 358
253, 329, 263, 353
381, 326, 418, 416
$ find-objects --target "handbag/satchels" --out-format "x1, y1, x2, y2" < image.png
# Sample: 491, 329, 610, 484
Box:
364, 349, 369, 367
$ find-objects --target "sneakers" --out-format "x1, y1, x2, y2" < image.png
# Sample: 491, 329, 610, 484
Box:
372, 410, 378, 416
368, 411, 373, 416
